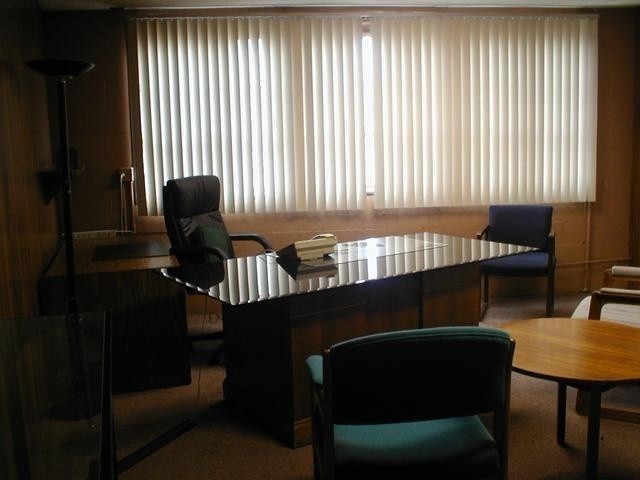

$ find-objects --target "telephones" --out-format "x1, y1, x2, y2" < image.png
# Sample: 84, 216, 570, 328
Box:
275, 233, 338, 261
278, 256, 339, 282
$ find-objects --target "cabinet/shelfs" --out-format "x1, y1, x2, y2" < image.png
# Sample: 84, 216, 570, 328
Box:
42, 238, 193, 395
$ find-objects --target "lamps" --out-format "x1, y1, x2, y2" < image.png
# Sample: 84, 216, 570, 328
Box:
26, 55, 103, 424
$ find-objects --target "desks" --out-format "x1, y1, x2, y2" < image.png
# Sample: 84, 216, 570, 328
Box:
492, 316, 639, 480
154, 229, 540, 450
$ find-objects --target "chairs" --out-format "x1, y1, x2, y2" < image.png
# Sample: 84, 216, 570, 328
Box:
475, 204, 557, 320
160, 175, 276, 365
568, 266, 639, 421
304, 325, 516, 480
